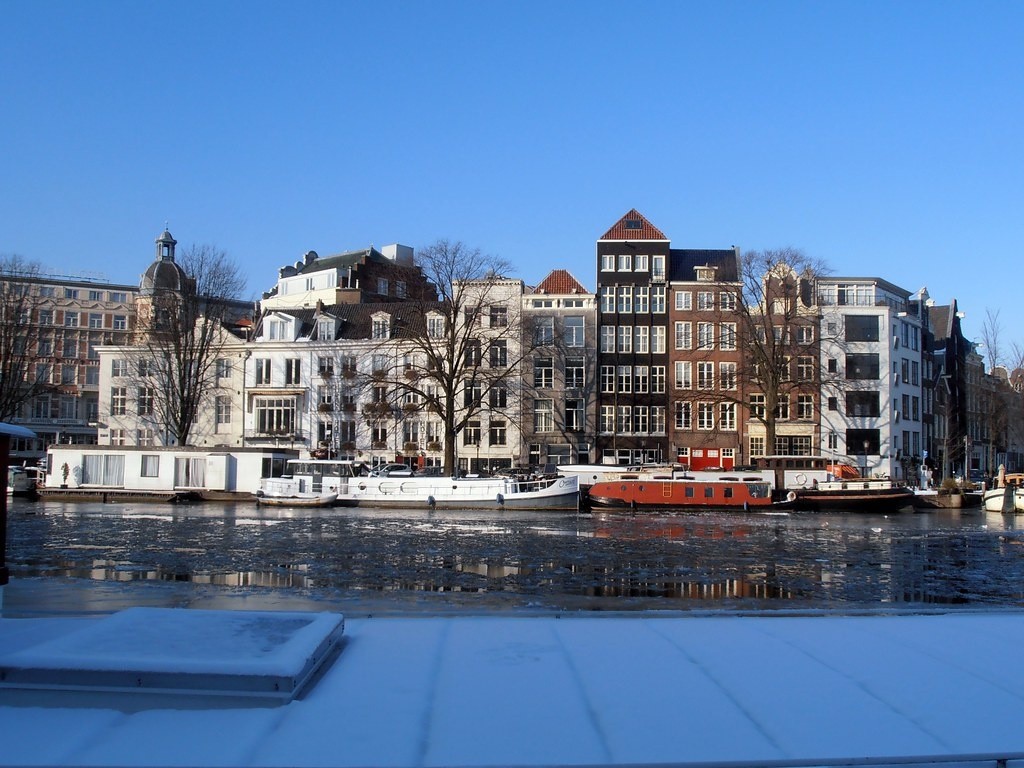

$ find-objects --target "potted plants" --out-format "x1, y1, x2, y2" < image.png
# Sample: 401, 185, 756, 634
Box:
61, 463, 69, 489
396, 451, 402, 456
419, 451, 425, 457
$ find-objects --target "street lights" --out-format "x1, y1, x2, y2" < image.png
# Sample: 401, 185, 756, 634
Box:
62, 425, 66, 444
864, 440, 870, 475
290, 435, 295, 450
641, 438, 646, 463
476, 437, 482, 469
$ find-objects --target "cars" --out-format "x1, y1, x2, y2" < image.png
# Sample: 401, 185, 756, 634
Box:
370, 463, 414, 475
495, 468, 529, 480
412, 466, 445, 477
970, 469, 990, 490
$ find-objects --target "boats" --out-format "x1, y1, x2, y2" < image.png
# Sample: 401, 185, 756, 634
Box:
256, 460, 581, 510
984, 464, 1024, 514
555, 432, 915, 513
175, 490, 257, 502
257, 490, 339, 509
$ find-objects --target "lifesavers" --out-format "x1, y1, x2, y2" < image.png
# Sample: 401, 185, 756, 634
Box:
795, 473, 807, 485
